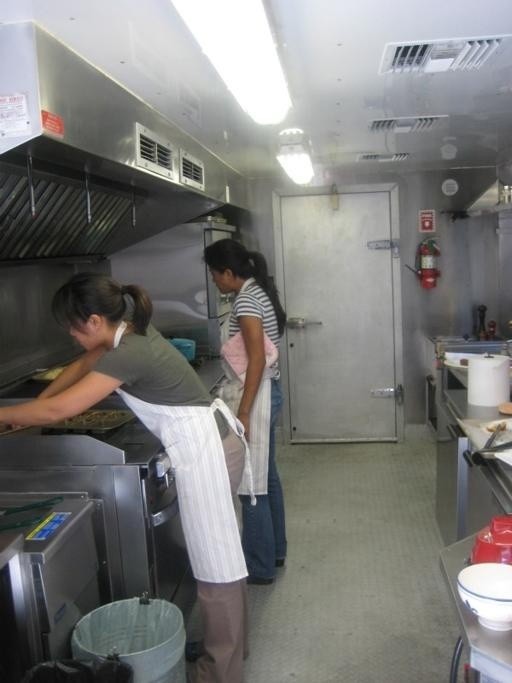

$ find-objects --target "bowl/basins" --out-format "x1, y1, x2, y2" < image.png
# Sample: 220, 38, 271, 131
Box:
454, 560, 512, 634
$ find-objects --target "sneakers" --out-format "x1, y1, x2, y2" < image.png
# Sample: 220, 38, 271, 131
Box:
182, 643, 203, 661
276, 559, 286, 567
246, 574, 274, 585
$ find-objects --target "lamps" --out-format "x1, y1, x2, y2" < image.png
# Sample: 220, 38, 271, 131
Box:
275, 131, 314, 185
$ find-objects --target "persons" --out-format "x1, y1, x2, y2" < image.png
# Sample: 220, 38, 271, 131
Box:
0, 272, 257, 682
200, 238, 289, 584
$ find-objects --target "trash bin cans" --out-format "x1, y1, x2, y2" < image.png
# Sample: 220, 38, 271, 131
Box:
23, 597, 188, 683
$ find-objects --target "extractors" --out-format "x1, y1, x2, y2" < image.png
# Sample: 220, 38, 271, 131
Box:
0, 46, 230, 267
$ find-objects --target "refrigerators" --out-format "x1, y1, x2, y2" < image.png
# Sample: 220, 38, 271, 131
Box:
101, 213, 238, 363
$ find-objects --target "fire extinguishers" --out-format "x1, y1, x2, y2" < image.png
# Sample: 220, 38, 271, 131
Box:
419, 236, 440, 288
